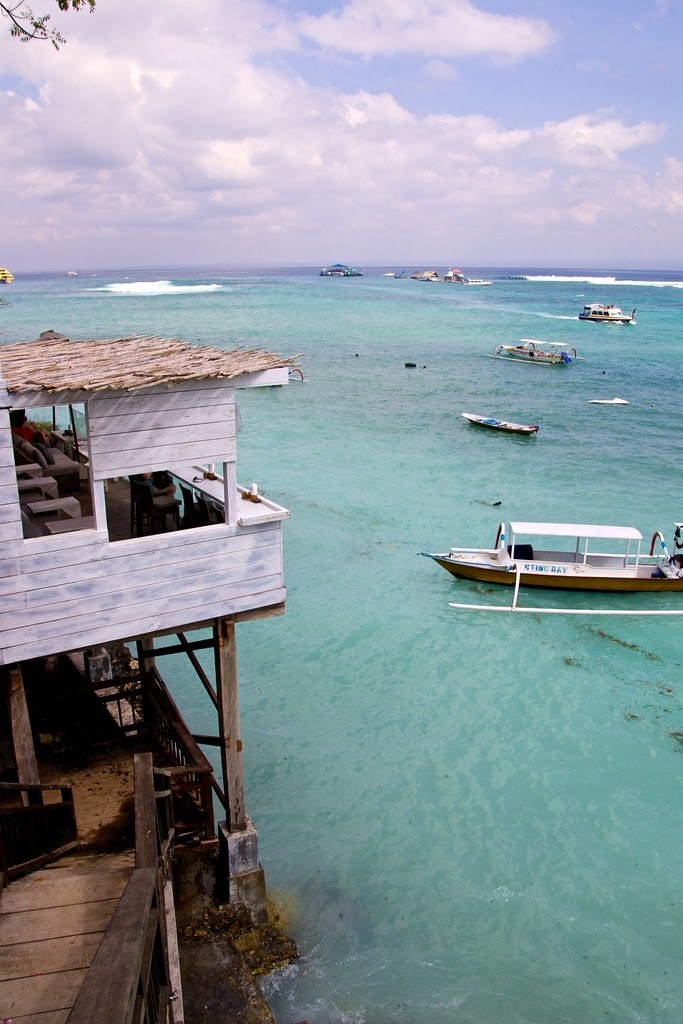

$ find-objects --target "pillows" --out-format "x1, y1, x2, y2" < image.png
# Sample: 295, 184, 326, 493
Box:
12, 433, 24, 449
32, 442, 56, 465
21, 439, 47, 469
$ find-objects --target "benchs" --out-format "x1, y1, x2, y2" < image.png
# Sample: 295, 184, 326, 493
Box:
13, 443, 81, 492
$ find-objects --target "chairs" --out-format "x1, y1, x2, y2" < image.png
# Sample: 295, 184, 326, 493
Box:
127, 475, 226, 536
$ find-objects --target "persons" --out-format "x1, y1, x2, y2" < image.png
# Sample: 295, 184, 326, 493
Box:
12, 409, 53, 448
134, 474, 176, 497
605, 305, 636, 318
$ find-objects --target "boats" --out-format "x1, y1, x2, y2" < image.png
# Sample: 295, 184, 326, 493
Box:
461, 278, 493, 286
461, 412, 539, 436
484, 337, 587, 366
395, 271, 408, 279
577, 302, 636, 324
416, 520, 683, 617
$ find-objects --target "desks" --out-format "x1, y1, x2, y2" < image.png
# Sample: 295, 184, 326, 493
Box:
51, 430, 119, 482
